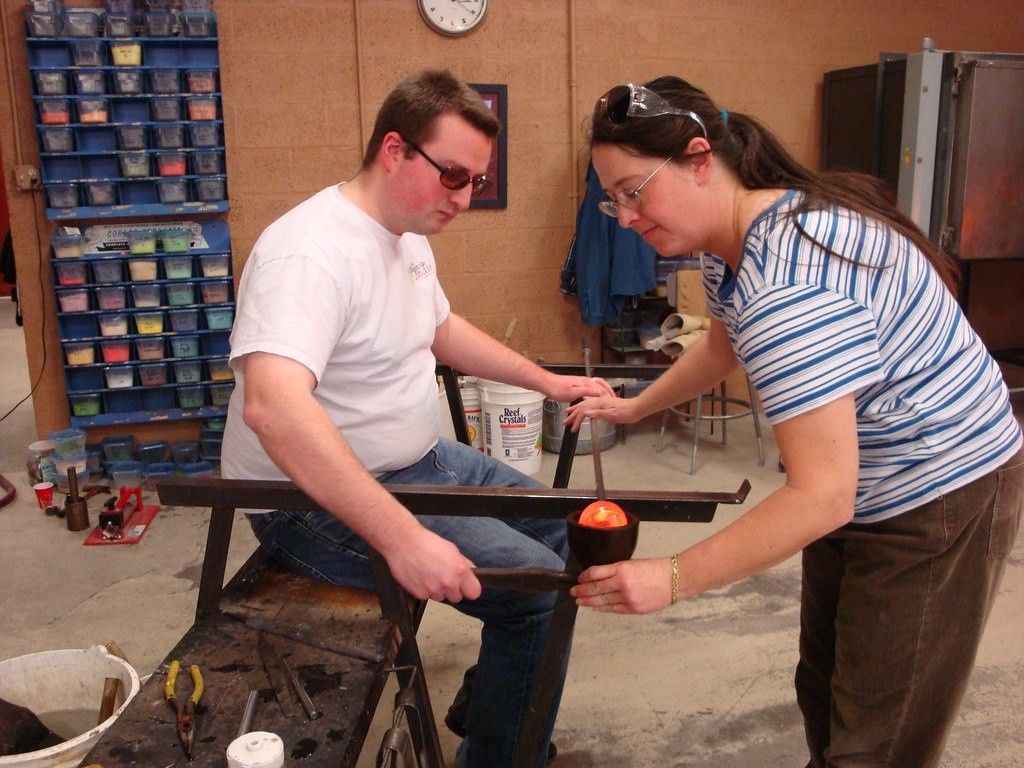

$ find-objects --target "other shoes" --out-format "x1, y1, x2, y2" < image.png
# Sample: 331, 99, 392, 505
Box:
444, 711, 557, 766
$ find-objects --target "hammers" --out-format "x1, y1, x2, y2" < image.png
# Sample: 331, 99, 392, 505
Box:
56, 485, 111, 518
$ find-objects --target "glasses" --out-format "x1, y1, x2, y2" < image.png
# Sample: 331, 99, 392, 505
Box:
399, 134, 490, 201
599, 152, 680, 217
593, 83, 708, 138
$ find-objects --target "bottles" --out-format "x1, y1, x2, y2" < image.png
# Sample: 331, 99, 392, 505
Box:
26, 440, 56, 481
225, 731, 287, 768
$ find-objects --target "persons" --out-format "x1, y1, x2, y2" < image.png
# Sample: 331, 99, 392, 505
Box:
220, 70, 617, 768
561, 75, 1024, 768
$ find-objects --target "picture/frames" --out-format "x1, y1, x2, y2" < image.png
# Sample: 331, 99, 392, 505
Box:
464, 84, 509, 211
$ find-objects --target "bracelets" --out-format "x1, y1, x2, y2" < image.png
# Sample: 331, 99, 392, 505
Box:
671, 554, 680, 604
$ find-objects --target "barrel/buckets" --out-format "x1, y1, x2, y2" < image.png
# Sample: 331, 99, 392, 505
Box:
437, 375, 616, 475
0, 644, 141, 768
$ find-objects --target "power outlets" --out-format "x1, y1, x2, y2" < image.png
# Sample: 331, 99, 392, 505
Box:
14, 166, 42, 193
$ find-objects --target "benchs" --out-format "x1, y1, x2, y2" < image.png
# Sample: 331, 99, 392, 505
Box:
75, 549, 446, 768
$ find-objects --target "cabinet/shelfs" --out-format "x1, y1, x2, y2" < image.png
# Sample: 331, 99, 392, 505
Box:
50, 226, 237, 478
417, 0, 490, 37
24, 5, 229, 221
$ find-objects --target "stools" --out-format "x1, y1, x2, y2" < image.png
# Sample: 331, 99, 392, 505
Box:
656, 338, 764, 474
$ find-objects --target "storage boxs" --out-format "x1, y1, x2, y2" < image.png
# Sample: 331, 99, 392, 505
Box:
188, 123, 221, 148
25, 12, 61, 38
40, 125, 71, 153
189, 152, 221, 173
74, 98, 109, 123
111, 67, 144, 93
29, 0, 215, 12
64, 10, 99, 39
118, 154, 151, 177
180, 12, 216, 38
45, 184, 235, 494
107, 39, 143, 67
604, 258, 702, 366
152, 125, 183, 148
185, 96, 218, 121
69, 38, 103, 69
115, 125, 148, 149
143, 10, 175, 37
32, 96, 69, 124
33, 69, 68, 96
102, 12, 137, 39
150, 97, 179, 120
184, 68, 216, 93
156, 152, 186, 176
149, 67, 180, 93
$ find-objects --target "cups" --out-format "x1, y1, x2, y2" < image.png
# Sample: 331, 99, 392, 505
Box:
33, 482, 54, 509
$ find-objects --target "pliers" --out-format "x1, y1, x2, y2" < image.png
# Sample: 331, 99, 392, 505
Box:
164, 660, 203, 757
